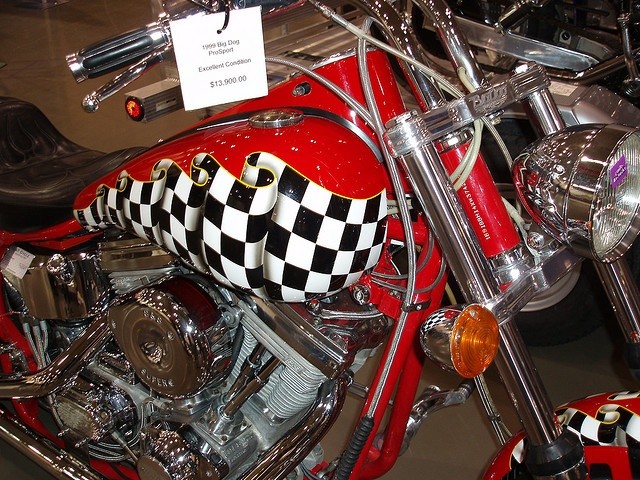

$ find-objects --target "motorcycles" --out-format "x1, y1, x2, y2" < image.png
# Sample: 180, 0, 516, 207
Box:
0, 0, 640, 480
